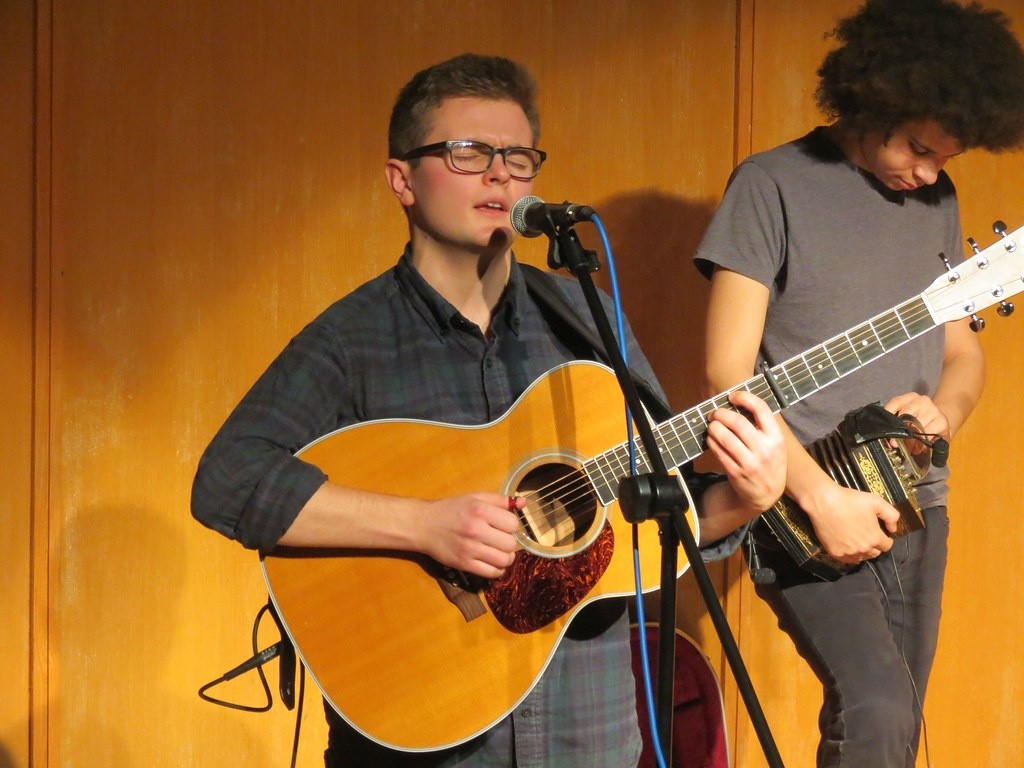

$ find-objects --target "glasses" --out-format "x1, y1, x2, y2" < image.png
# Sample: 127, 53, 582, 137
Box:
399, 140, 547, 179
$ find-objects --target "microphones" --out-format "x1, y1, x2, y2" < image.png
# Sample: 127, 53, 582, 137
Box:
746, 532, 776, 586
852, 431, 949, 469
510, 195, 597, 238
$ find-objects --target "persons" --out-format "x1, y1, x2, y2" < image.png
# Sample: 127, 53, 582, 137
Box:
692, 1, 1024, 768
190, 53, 788, 768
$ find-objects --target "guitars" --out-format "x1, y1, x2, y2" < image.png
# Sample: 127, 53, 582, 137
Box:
257, 217, 1024, 754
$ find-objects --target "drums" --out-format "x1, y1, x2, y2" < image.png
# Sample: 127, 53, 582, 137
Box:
760, 402, 927, 583
